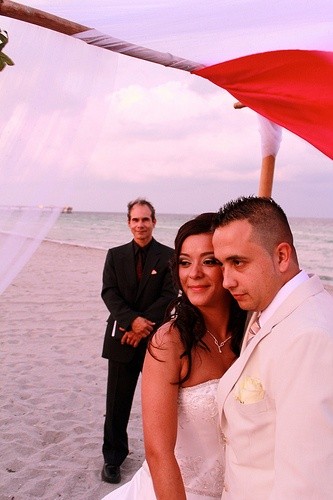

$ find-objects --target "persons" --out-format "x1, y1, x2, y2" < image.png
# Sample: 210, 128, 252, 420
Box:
100, 199, 180, 484
139, 212, 237, 498
207, 199, 331, 495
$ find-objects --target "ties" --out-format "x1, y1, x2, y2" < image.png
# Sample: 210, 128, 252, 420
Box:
247, 321, 261, 347
137, 253, 143, 283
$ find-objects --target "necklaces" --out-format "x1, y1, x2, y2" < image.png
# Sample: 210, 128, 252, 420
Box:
202, 327, 239, 354
